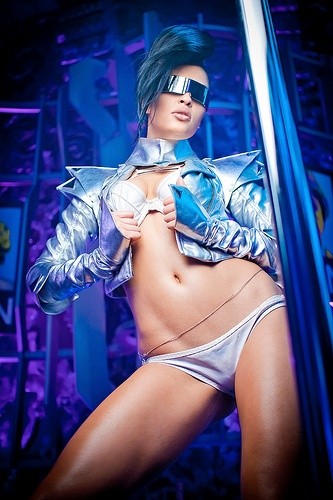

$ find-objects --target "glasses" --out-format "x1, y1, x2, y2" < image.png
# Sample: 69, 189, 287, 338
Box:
156, 74, 210, 109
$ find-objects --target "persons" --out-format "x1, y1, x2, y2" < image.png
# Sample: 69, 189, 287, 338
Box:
29, 24, 302, 500
